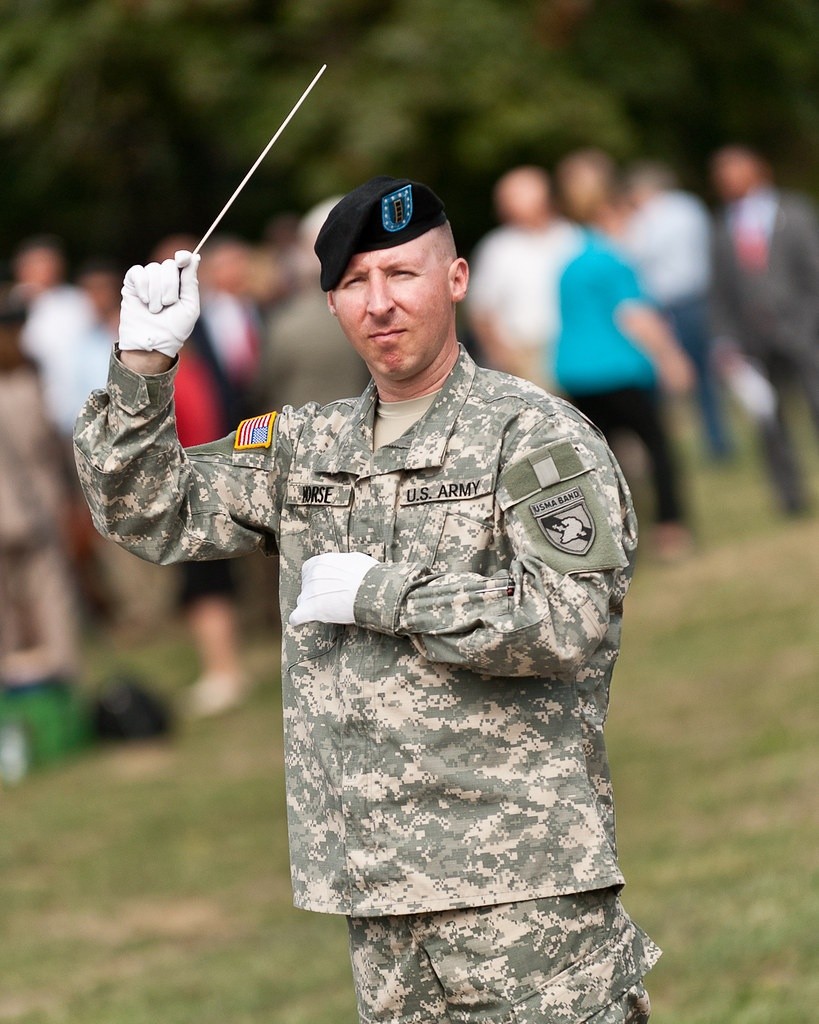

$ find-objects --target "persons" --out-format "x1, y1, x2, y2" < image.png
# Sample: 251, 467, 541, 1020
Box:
0, 143, 819, 782
70, 179, 663, 1024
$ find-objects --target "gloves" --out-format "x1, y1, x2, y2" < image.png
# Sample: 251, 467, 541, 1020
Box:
288, 551, 381, 627
117, 250, 200, 359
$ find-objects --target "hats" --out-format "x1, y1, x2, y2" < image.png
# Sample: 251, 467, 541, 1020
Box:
314, 174, 448, 292
